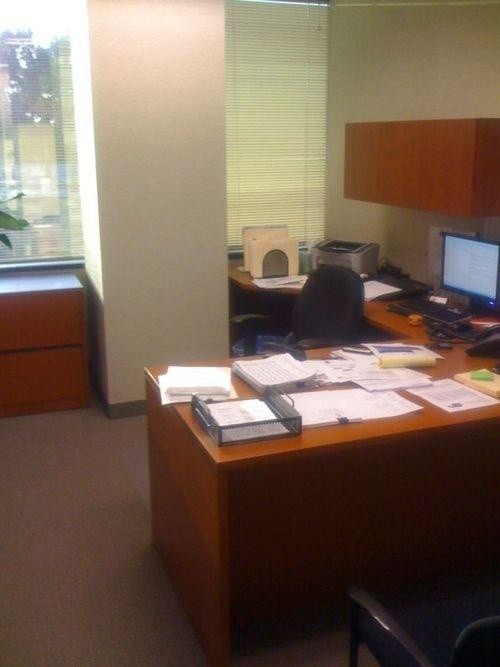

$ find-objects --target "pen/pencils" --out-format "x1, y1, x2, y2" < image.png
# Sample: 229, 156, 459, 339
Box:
343, 349, 370, 354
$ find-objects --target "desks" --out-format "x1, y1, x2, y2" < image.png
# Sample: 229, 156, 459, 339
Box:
143, 254, 500, 666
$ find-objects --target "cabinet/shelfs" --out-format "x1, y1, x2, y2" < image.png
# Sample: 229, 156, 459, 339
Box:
343, 118, 500, 218
1, 271, 90, 417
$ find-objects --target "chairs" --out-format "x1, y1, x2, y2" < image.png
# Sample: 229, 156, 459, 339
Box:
230, 263, 365, 355
346, 582, 500, 666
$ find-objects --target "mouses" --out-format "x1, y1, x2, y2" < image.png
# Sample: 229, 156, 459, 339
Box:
432, 328, 456, 339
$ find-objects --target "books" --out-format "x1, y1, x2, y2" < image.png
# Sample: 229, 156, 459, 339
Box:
166, 366, 233, 395
454, 367, 500, 398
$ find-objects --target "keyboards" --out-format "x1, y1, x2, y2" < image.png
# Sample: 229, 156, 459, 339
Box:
384, 294, 472, 331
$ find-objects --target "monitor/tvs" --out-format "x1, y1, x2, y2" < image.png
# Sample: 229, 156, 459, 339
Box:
440, 232, 500, 314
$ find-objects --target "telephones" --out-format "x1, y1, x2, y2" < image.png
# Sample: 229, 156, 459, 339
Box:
464, 324, 500, 355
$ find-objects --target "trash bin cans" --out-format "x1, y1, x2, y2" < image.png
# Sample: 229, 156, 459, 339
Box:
232, 334, 282, 355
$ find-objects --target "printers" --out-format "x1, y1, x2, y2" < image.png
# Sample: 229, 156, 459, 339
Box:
311, 238, 380, 279
